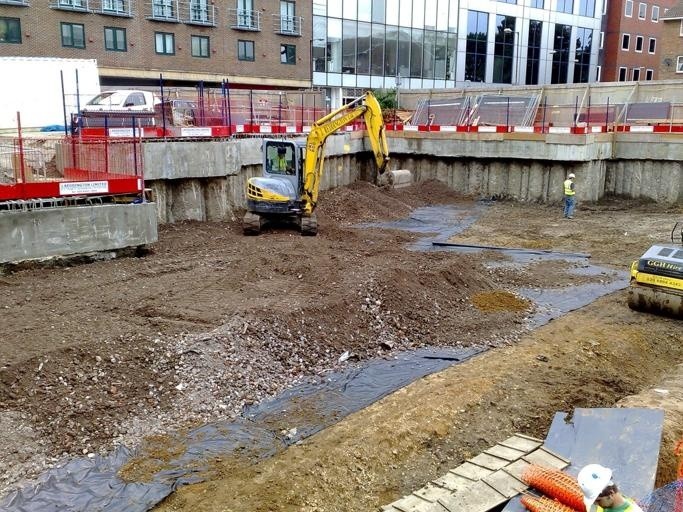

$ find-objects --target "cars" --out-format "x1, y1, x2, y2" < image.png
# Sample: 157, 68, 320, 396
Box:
82, 91, 163, 127
156, 100, 224, 127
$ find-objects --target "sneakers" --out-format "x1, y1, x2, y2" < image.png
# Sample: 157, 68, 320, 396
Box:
563, 214, 575, 219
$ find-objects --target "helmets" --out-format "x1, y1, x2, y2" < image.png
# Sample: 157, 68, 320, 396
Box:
568, 173, 575, 179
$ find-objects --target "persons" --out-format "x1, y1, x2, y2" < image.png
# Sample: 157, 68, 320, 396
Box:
562, 171, 577, 220
576, 464, 642, 512
271, 145, 294, 175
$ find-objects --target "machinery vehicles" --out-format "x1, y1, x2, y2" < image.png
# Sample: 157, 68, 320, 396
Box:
240, 90, 413, 237
626, 245, 683, 319
